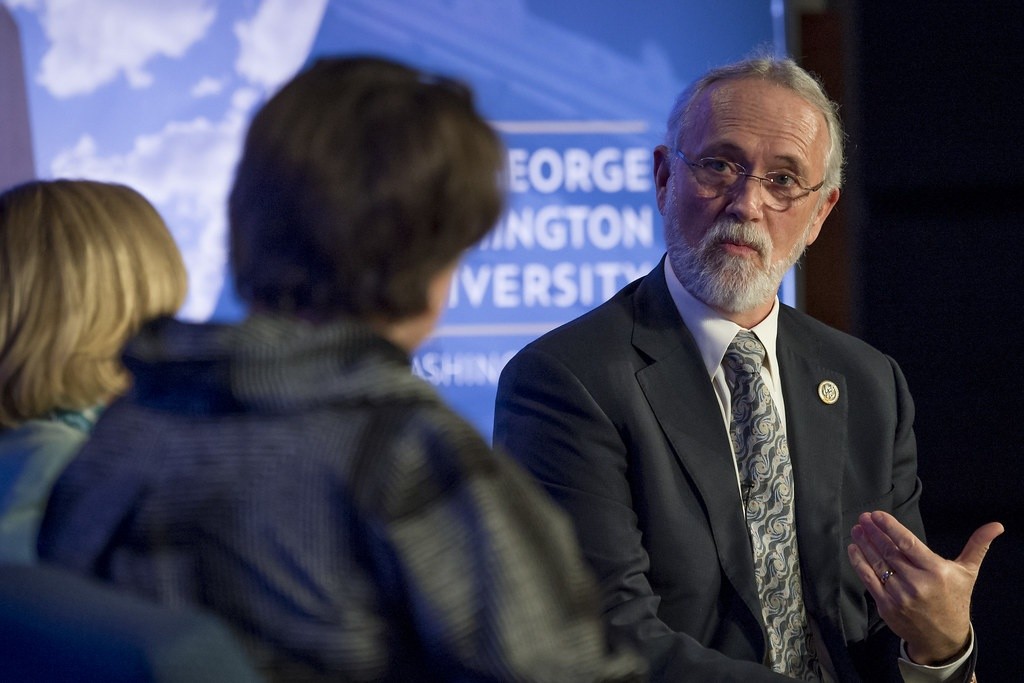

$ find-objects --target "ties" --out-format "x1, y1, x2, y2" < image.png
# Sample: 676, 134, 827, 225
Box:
721, 329, 824, 682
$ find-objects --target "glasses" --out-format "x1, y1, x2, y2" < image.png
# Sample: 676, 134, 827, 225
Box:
675, 150, 825, 207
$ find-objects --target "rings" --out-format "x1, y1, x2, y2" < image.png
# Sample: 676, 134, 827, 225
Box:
881, 569, 894, 584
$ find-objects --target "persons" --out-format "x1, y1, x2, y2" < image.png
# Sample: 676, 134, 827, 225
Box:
40, 54, 648, 683
492, 58, 1004, 683
0, 177, 187, 572
